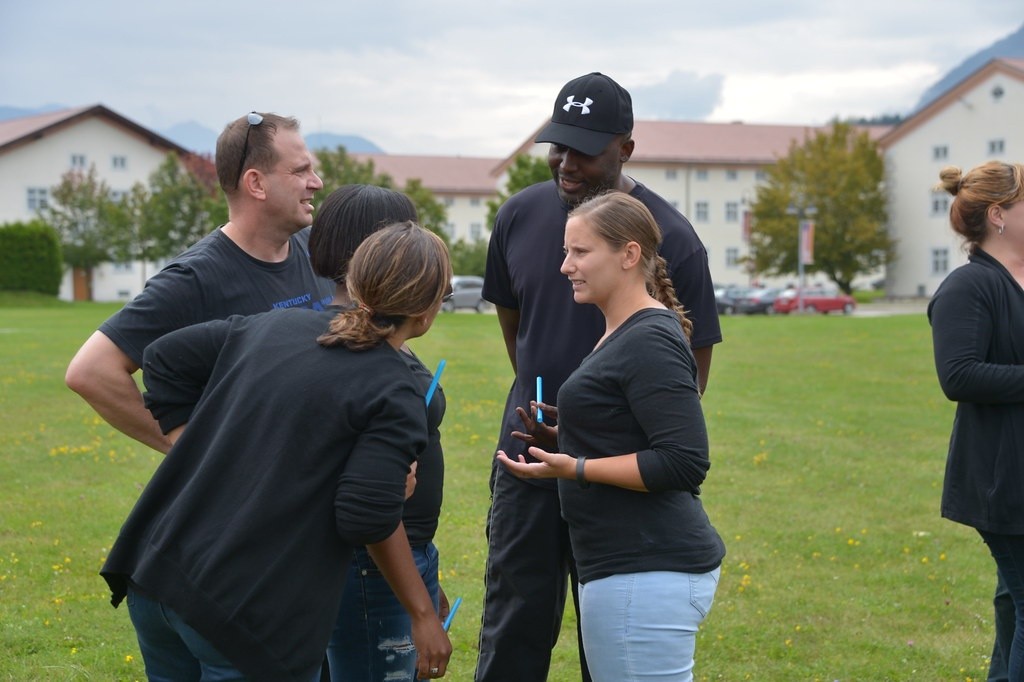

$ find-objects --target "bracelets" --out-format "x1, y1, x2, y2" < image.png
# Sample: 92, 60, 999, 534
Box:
576, 456, 591, 489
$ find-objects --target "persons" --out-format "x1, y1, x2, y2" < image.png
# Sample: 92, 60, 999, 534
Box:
99, 220, 454, 682
64, 111, 336, 456
308, 184, 451, 682
474, 71, 723, 682
496, 190, 726, 682
926, 161, 1024, 682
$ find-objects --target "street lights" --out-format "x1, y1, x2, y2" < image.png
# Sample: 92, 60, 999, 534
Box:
785, 193, 818, 315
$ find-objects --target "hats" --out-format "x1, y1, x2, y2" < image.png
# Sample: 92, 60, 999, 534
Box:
533, 72, 634, 156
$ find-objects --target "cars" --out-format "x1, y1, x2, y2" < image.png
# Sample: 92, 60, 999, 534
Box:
440, 277, 487, 314
714, 289, 740, 316
774, 284, 855, 316
734, 287, 783, 316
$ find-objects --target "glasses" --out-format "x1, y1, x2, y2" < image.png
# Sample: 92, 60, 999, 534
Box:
440, 284, 454, 303
234, 110, 264, 191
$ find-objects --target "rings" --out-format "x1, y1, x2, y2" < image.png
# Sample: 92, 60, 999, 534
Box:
430, 667, 439, 674
